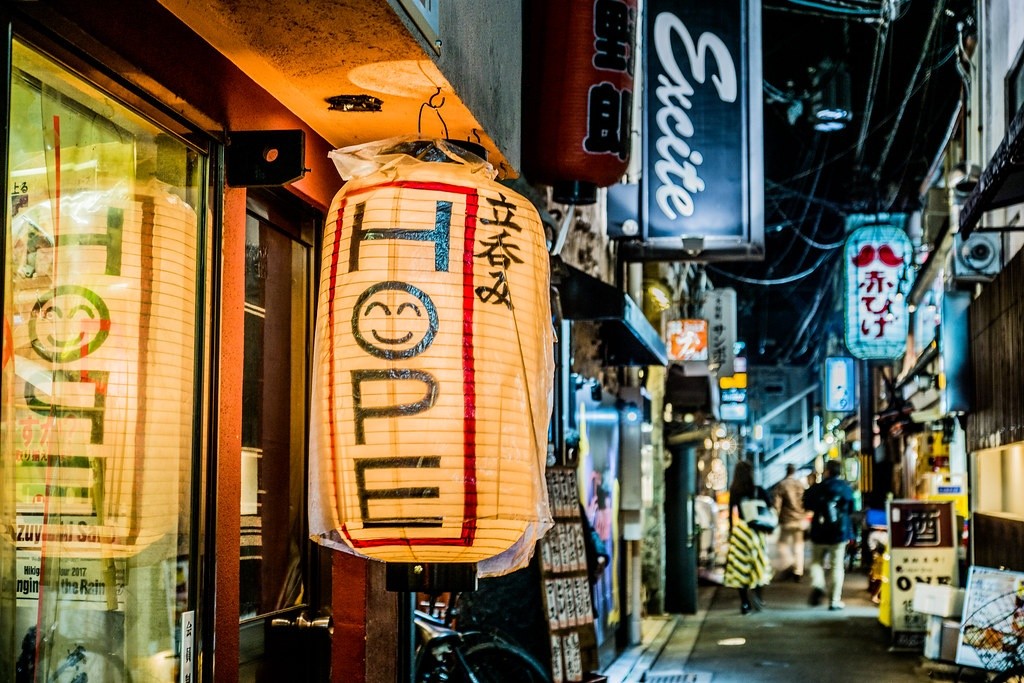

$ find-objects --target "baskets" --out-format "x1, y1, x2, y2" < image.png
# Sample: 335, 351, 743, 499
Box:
960, 588, 1024, 671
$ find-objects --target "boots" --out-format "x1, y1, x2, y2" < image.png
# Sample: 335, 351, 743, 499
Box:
736, 585, 752, 614
750, 586, 765, 608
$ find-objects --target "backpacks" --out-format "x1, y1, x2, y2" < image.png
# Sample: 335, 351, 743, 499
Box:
815, 483, 847, 539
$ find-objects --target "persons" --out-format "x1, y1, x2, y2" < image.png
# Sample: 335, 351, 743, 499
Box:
723, 461, 778, 613
694, 486, 720, 558
800, 460, 855, 608
773, 461, 812, 582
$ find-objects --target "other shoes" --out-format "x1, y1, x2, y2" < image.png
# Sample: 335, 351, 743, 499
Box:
781, 566, 795, 578
793, 573, 802, 583
808, 586, 825, 607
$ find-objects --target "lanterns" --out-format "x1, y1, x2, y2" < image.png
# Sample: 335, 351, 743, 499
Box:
520, 0, 643, 206
301, 132, 555, 560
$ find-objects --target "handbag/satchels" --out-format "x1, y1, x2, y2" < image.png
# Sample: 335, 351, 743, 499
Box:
740, 485, 778, 533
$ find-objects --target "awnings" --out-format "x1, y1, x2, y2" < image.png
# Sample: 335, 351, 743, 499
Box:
836, 402, 915, 430
894, 337, 939, 389
960, 103, 1024, 242
559, 262, 667, 368
666, 357, 723, 422
